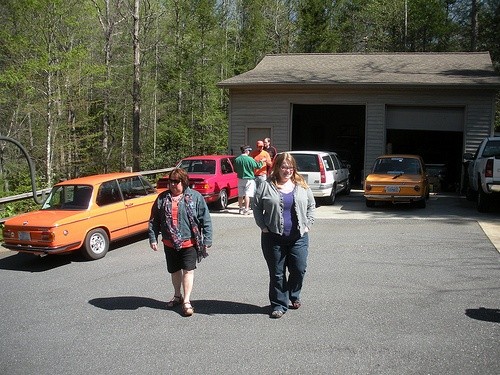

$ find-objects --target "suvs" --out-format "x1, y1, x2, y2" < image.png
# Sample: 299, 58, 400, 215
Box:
269, 151, 351, 206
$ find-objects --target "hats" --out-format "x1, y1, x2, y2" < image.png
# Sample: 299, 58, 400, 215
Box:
257, 141, 264, 146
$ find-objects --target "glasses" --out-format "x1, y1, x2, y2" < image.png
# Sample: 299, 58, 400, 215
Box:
280, 165, 296, 170
168, 178, 181, 183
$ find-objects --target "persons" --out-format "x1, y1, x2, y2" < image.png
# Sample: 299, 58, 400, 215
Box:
249, 140, 273, 210
253, 152, 316, 318
148, 168, 212, 316
234, 145, 264, 215
263, 137, 277, 180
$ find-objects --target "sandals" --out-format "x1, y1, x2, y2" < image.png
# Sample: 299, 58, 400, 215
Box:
168, 295, 182, 308
182, 302, 193, 315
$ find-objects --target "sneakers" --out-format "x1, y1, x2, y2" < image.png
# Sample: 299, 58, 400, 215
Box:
240, 208, 253, 215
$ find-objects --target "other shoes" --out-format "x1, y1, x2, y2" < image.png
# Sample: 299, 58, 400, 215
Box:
271, 310, 283, 318
292, 299, 302, 308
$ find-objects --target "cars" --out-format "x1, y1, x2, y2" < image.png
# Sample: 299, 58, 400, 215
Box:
0, 172, 169, 259
362, 154, 430, 209
156, 154, 242, 210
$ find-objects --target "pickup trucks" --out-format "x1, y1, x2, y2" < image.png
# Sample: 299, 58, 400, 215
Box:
463, 136, 500, 213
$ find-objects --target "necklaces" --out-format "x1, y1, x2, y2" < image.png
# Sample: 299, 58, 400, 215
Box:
172, 193, 183, 202
